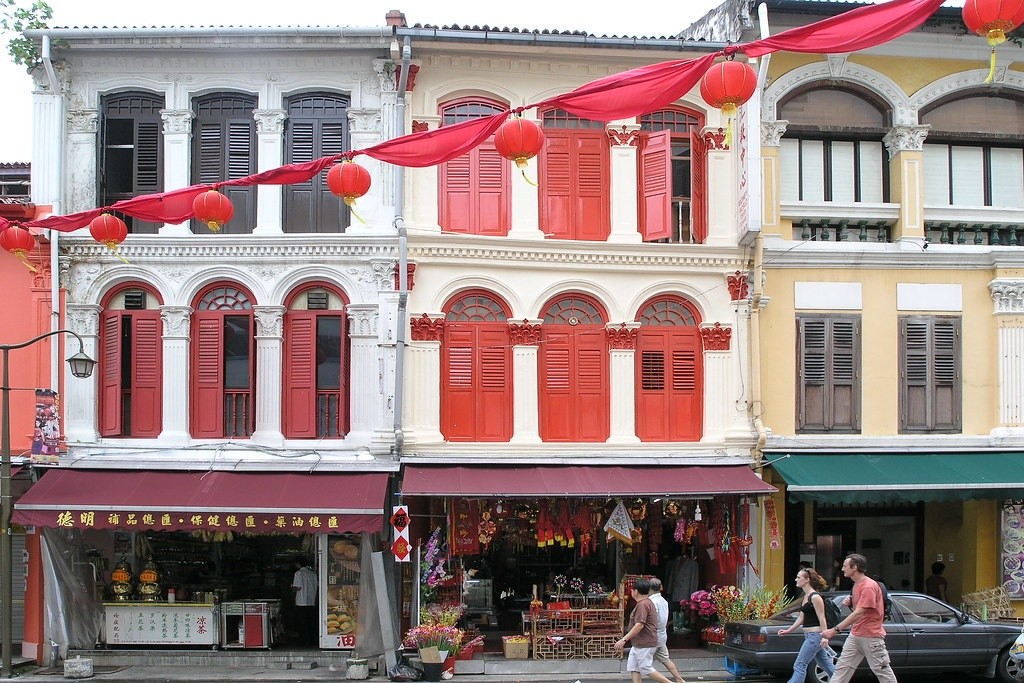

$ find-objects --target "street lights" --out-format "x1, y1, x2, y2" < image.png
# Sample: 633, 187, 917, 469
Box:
1, 330, 97, 676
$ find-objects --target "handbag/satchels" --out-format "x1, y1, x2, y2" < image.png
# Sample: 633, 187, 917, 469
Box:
848, 575, 892, 622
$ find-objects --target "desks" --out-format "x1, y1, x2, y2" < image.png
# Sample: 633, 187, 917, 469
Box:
521, 612, 547, 636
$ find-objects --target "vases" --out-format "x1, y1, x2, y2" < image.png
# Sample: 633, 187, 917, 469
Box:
424, 656, 454, 682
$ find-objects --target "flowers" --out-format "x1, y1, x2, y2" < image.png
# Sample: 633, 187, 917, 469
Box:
402, 525, 488, 662
709, 585, 795, 633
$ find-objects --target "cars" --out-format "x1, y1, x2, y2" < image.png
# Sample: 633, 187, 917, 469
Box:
723, 590, 1024, 683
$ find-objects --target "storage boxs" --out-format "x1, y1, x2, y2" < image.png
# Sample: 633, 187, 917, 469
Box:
503, 639, 529, 659
457, 651, 472, 660
473, 646, 484, 653
725, 657, 760, 676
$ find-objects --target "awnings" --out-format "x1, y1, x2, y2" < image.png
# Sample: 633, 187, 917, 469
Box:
10, 466, 387, 534
399, 459, 779, 494
762, 449, 1021, 492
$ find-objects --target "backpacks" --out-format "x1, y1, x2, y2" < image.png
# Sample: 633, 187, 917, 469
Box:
808, 591, 842, 629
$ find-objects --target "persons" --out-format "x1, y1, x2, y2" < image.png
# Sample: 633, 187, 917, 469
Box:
778, 567, 836, 683
647, 577, 686, 683
290, 556, 317, 651
539, 570, 554, 601
926, 562, 948, 603
614, 581, 673, 683
819, 554, 898, 683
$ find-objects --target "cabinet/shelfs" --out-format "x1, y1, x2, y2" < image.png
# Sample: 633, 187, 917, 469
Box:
147, 541, 313, 603
72, 549, 106, 650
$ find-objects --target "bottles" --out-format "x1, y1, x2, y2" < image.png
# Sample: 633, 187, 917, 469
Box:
168, 589, 176, 604
192, 592, 214, 604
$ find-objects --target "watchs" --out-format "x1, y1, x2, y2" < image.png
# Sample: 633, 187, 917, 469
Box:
834, 626, 840, 633
621, 637, 627, 642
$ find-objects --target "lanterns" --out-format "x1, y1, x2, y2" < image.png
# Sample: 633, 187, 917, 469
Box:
494, 117, 544, 186
700, 59, 757, 146
193, 189, 233, 233
327, 156, 371, 223
962, 0, 1024, 85
90, 212, 129, 264
0, 223, 40, 273
